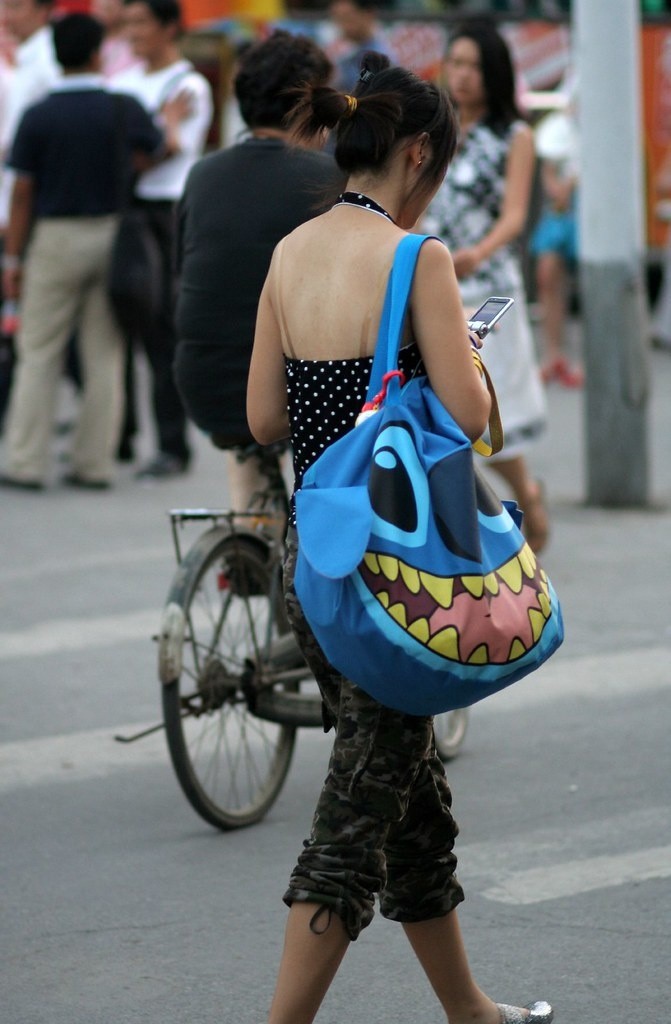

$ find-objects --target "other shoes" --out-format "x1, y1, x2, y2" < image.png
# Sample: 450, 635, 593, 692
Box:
66, 476, 108, 489
139, 457, 187, 480
496, 1000, 554, 1023
2, 476, 40, 490
538, 360, 581, 390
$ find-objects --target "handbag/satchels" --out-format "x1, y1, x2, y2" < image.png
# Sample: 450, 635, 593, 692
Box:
293, 232, 566, 713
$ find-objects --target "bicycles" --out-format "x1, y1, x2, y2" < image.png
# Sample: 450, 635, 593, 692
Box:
114, 430, 471, 828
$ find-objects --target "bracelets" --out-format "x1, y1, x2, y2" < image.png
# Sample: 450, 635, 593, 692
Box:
469, 335, 478, 349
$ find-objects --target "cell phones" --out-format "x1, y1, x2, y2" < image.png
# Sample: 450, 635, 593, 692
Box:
465, 296, 513, 339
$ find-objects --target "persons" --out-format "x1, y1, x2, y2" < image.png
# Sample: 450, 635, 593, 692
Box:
172, 34, 347, 602
329, 1, 394, 92
247, 67, 554, 1024
537, 85, 578, 389
1, 0, 215, 490
407, 24, 549, 553
